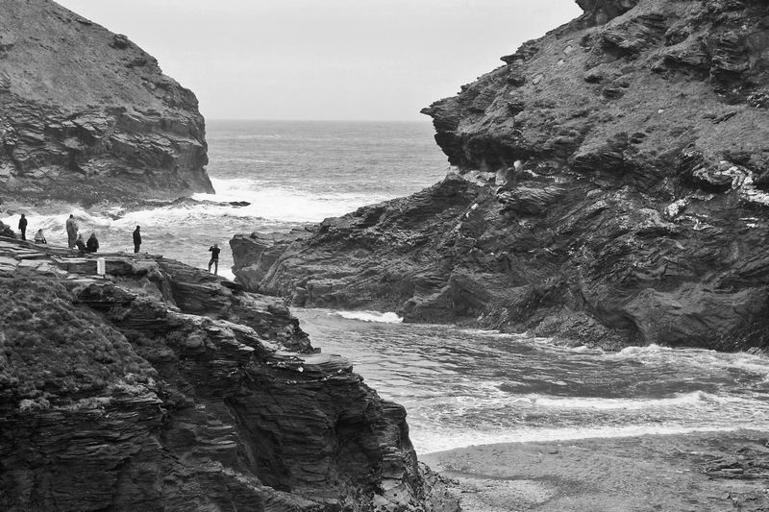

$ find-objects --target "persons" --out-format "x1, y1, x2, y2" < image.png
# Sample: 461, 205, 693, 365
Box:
18, 214, 27, 239
87, 233, 99, 252
208, 244, 220, 274
133, 225, 142, 253
66, 213, 77, 248
34, 228, 46, 243
76, 235, 86, 251
